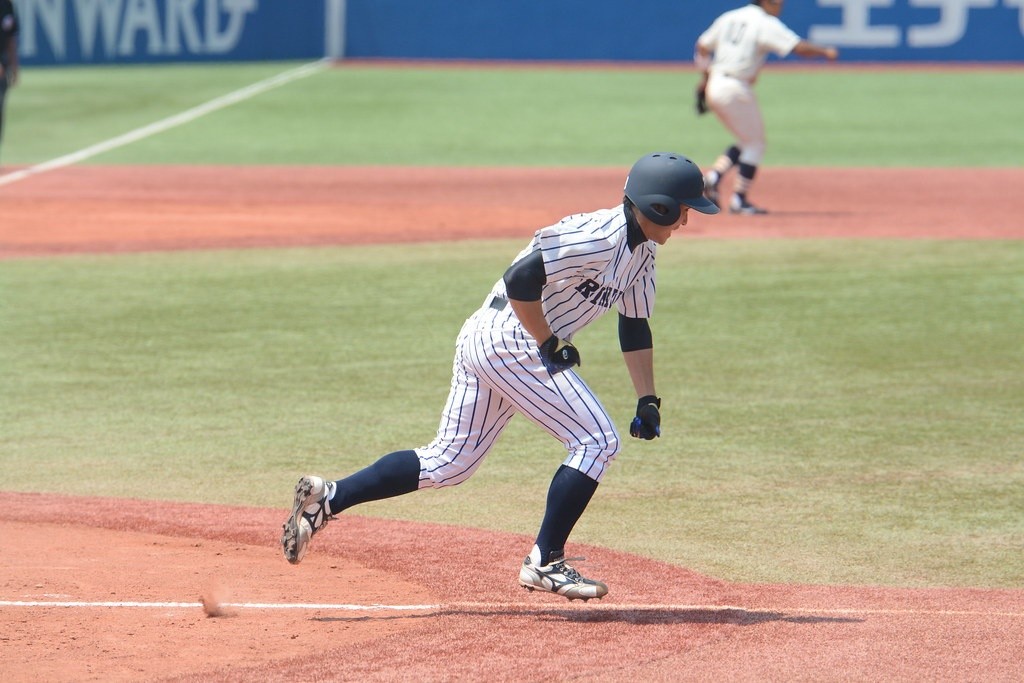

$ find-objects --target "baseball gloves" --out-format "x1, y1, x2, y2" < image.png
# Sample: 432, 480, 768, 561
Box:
694, 70, 709, 115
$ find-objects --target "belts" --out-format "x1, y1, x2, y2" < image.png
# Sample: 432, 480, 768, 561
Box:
489, 296, 506, 310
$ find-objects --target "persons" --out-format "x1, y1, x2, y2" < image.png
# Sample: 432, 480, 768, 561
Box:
280, 152, 720, 603
694, 0, 838, 216
0, 0, 19, 132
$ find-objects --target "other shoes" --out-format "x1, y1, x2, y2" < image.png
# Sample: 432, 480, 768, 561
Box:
703, 171, 721, 208
728, 192, 766, 215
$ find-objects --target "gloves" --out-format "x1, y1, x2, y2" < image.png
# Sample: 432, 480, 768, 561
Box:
539, 334, 580, 374
629, 395, 662, 440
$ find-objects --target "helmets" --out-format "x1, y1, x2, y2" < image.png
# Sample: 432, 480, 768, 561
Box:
623, 152, 720, 226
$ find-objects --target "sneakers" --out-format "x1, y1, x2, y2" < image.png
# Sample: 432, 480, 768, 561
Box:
281, 476, 339, 564
518, 544, 608, 602
